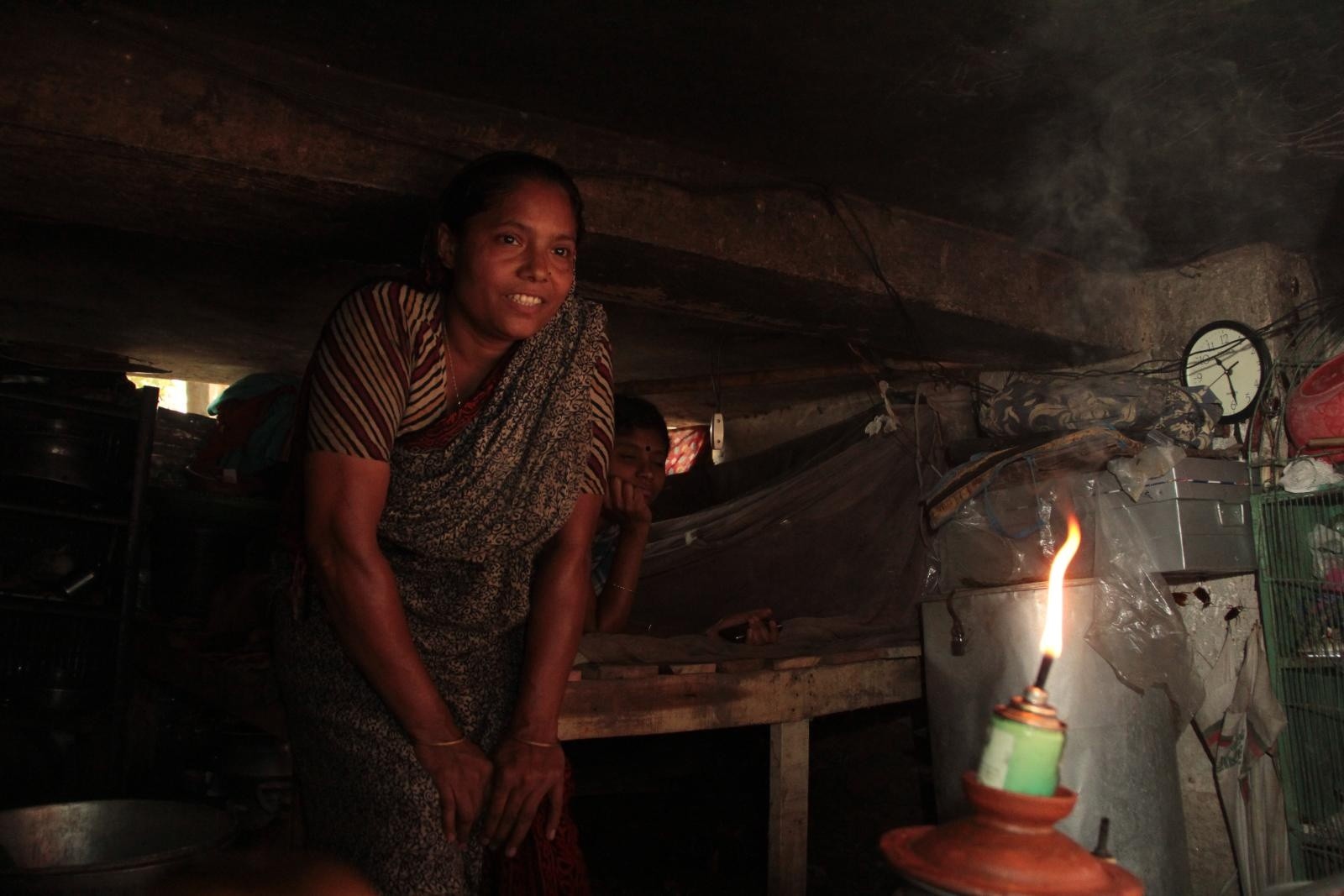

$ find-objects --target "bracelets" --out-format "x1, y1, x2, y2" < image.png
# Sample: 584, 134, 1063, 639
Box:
510, 735, 558, 747
416, 734, 467, 747
605, 579, 639, 596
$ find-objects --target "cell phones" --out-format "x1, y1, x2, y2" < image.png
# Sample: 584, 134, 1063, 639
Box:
719, 616, 785, 644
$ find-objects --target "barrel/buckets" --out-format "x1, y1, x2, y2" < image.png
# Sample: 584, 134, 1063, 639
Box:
916, 576, 1193, 896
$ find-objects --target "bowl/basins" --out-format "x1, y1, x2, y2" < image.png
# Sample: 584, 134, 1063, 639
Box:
0, 798, 235, 896
0, 417, 102, 713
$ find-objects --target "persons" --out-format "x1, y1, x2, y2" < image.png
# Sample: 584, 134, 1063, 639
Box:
271, 151, 779, 895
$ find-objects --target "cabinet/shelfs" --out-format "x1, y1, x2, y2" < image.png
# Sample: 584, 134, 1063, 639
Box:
0, 375, 157, 811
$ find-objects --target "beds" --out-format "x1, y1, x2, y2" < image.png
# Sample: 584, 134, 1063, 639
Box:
555, 639, 922, 896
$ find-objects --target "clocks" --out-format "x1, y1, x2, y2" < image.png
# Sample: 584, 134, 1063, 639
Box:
1179, 321, 1273, 424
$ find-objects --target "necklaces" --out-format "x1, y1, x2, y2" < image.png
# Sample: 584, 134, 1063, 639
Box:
441, 319, 464, 409
